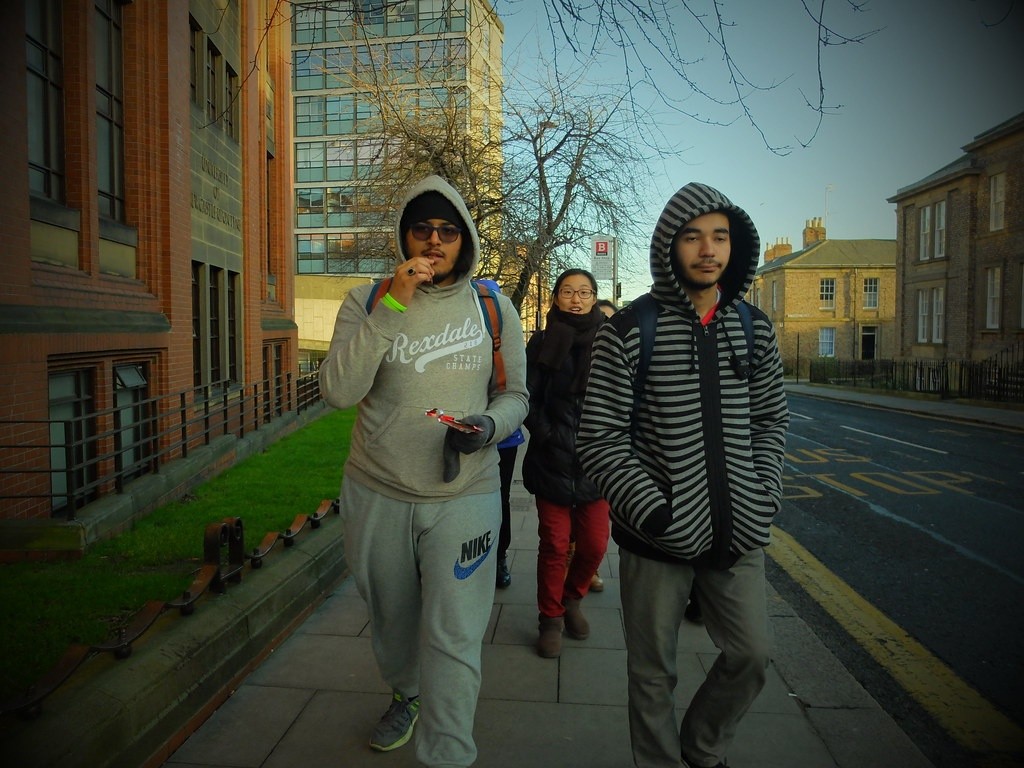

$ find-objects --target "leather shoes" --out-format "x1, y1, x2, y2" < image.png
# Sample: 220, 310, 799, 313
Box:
495, 552, 511, 586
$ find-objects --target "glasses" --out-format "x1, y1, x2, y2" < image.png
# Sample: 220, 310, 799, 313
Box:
555, 288, 597, 299
410, 222, 462, 242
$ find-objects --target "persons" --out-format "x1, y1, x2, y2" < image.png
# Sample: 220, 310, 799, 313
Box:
575, 183, 790, 768
319, 175, 529, 768
473, 279, 525, 589
522, 269, 610, 659
564, 300, 619, 593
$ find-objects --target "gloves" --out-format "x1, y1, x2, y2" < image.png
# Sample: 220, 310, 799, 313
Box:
442, 427, 460, 483
453, 414, 493, 455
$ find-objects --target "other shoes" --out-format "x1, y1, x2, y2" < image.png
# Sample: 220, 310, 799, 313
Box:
683, 588, 706, 625
588, 569, 604, 592
561, 588, 591, 640
563, 542, 575, 582
537, 612, 565, 657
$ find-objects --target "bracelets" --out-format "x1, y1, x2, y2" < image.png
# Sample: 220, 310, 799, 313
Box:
382, 293, 408, 313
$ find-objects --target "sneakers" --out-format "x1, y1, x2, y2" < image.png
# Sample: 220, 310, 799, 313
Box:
369, 688, 420, 751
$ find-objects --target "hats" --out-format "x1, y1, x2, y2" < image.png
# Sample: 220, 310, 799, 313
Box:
400, 190, 474, 274
